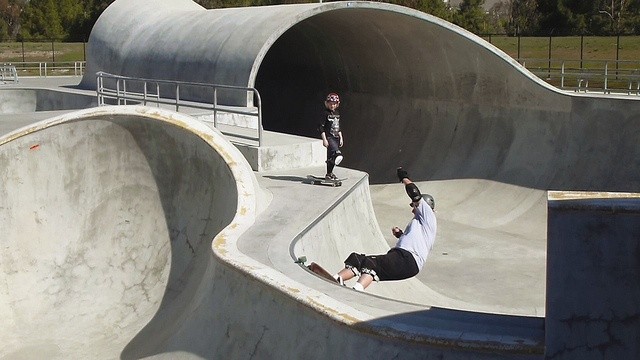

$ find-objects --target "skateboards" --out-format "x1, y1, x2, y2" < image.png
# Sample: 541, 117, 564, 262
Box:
307, 174, 348, 187
298, 256, 339, 283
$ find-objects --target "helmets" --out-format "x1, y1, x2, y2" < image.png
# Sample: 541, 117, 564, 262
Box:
410, 194, 434, 209
326, 93, 340, 102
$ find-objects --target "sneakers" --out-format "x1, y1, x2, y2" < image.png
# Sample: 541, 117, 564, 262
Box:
325, 173, 339, 181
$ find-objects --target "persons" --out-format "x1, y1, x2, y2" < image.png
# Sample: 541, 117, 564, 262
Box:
332, 167, 436, 293
318, 94, 343, 181
